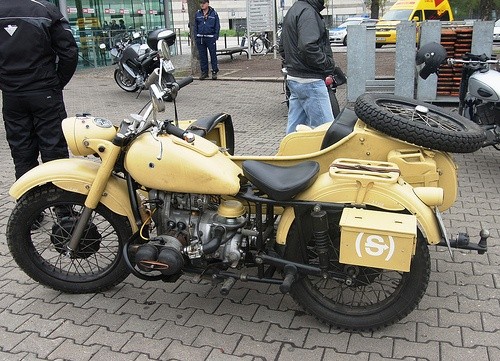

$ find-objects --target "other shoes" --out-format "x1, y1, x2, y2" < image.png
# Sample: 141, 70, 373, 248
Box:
57, 211, 81, 222
199, 72, 209, 79
212, 73, 217, 80
30, 213, 44, 230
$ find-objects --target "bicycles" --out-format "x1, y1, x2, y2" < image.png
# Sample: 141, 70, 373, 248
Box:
238, 22, 264, 56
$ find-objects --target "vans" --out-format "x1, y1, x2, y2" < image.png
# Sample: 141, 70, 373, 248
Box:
375, 0, 453, 48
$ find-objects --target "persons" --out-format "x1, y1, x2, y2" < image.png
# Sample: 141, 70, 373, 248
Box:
91, 19, 127, 66
0, 0, 79, 231
194, 0, 220, 80
278, 0, 340, 136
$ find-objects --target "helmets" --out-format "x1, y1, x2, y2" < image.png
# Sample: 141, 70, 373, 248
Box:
415, 41, 448, 80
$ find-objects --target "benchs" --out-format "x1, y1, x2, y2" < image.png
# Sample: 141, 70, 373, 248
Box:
216, 47, 249, 64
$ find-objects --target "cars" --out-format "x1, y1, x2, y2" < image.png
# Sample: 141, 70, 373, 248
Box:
493, 19, 500, 43
326, 18, 379, 46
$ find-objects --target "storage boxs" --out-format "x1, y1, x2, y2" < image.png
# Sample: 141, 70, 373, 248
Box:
147, 28, 176, 52
338, 208, 417, 272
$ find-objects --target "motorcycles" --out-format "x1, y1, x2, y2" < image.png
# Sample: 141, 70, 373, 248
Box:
281, 43, 347, 119
7, 16, 485, 331
415, 41, 500, 152
99, 23, 179, 101
